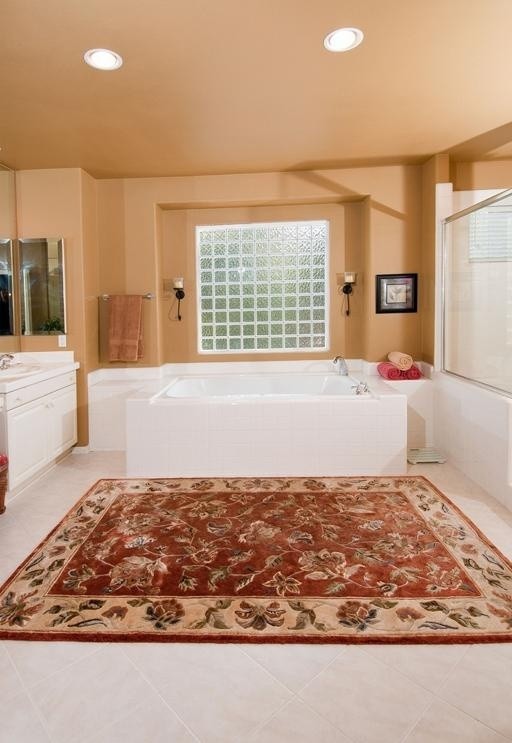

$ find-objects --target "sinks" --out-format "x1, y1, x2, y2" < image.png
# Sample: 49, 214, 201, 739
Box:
0, 361, 44, 378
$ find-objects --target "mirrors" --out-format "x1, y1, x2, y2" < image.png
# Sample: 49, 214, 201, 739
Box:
18, 234, 68, 336
1, 160, 18, 336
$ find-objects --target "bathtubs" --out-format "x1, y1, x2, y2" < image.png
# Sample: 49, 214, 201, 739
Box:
127, 358, 408, 479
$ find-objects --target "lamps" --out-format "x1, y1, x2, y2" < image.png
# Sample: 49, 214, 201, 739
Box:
342, 271, 356, 318
173, 277, 186, 321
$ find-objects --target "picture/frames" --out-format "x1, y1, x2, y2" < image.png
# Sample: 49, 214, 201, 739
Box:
375, 274, 419, 314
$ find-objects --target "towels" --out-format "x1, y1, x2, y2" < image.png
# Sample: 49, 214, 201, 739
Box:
108, 294, 144, 363
375, 350, 422, 381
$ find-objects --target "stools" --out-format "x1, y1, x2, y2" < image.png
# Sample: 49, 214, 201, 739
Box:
0, 454, 13, 514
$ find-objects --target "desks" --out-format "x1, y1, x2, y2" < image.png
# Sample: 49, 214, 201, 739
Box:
0, 358, 81, 505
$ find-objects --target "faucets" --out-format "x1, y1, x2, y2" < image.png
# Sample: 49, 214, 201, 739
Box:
333, 355, 350, 376
0, 352, 16, 362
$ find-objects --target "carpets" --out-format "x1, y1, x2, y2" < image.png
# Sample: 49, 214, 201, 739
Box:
0, 474, 512, 646
